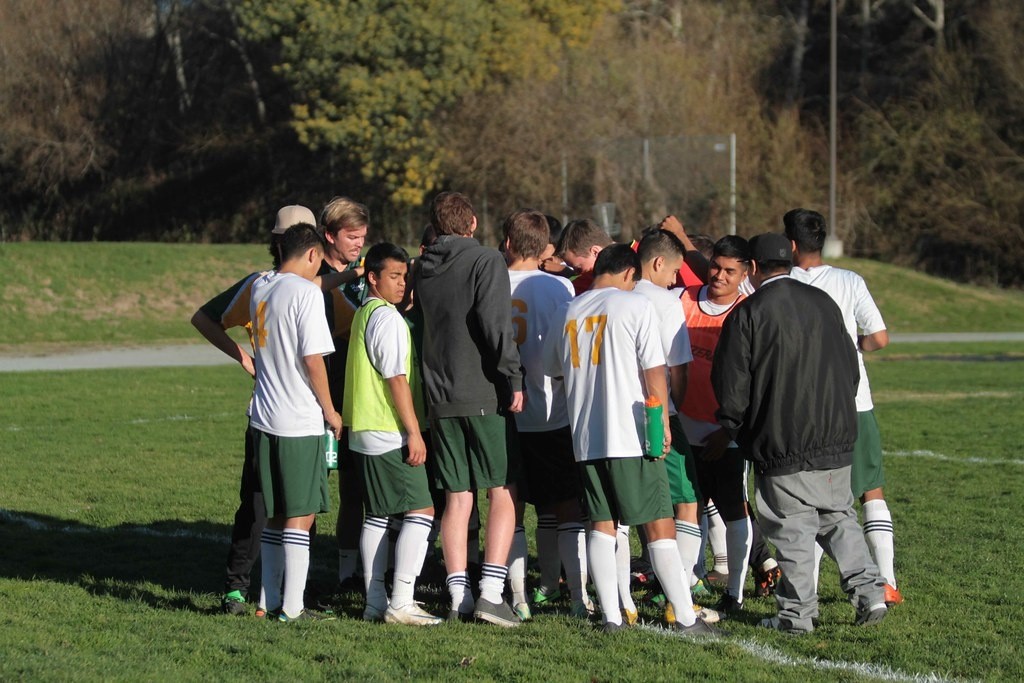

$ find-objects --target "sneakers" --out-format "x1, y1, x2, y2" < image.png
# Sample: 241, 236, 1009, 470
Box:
852, 602, 886, 625
221, 594, 252, 615
665, 602, 727, 624
883, 583, 903, 606
761, 616, 805, 633
305, 580, 332, 610
362, 603, 385, 621
279, 611, 328, 624
690, 579, 725, 606
255, 606, 282, 621
474, 598, 519, 628
383, 603, 445, 624
514, 603, 533, 621
712, 593, 743, 614
535, 583, 638, 634
342, 572, 365, 596
755, 558, 778, 596
675, 618, 730, 636
447, 611, 473, 621
632, 559, 653, 589
651, 591, 665, 607
705, 569, 729, 590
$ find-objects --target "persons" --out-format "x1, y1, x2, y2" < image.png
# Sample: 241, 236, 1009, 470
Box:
190, 204, 323, 617
221, 223, 366, 623
782, 208, 903, 610
310, 195, 370, 606
710, 233, 888, 633
405, 189, 780, 639
341, 242, 446, 625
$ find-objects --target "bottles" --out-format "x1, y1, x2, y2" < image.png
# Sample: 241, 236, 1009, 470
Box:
324, 421, 339, 469
643, 394, 666, 457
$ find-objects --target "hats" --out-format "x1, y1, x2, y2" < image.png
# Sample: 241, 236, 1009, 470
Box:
747, 233, 795, 261
272, 205, 317, 234
